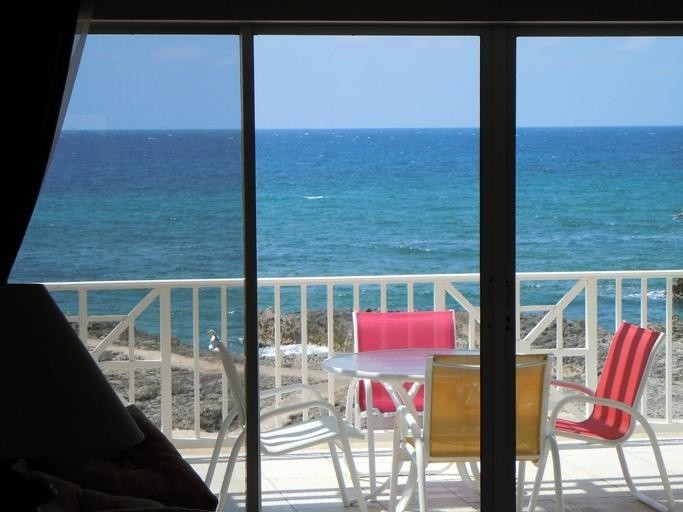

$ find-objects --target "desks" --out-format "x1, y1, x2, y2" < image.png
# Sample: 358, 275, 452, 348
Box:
319, 349, 542, 512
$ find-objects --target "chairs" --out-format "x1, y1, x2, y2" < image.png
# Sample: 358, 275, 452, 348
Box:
352, 309, 457, 504
205, 328, 368, 512
391, 353, 553, 512
518, 320, 674, 512
